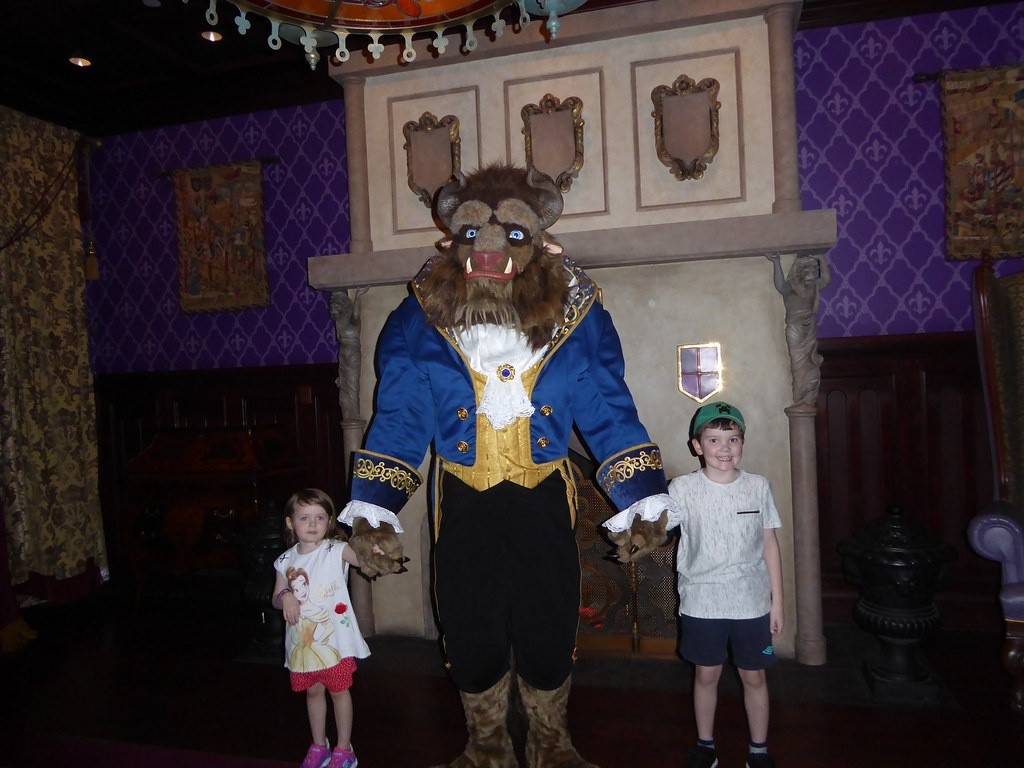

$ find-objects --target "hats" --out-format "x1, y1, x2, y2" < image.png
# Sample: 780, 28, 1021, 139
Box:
693, 402, 746, 437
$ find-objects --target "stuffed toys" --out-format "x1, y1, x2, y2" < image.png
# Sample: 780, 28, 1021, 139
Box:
337, 162, 668, 768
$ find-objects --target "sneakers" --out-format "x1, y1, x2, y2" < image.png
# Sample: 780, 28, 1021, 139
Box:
299, 737, 331, 768
327, 742, 358, 768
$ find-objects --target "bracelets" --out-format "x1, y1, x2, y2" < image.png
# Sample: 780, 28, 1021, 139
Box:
276, 588, 292, 605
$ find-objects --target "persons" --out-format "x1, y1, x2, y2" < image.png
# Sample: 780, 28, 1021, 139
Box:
666, 402, 784, 768
765, 252, 830, 412
271, 489, 385, 768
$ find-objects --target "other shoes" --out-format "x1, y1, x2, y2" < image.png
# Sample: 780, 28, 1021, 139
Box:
687, 745, 718, 768
746, 751, 774, 768
